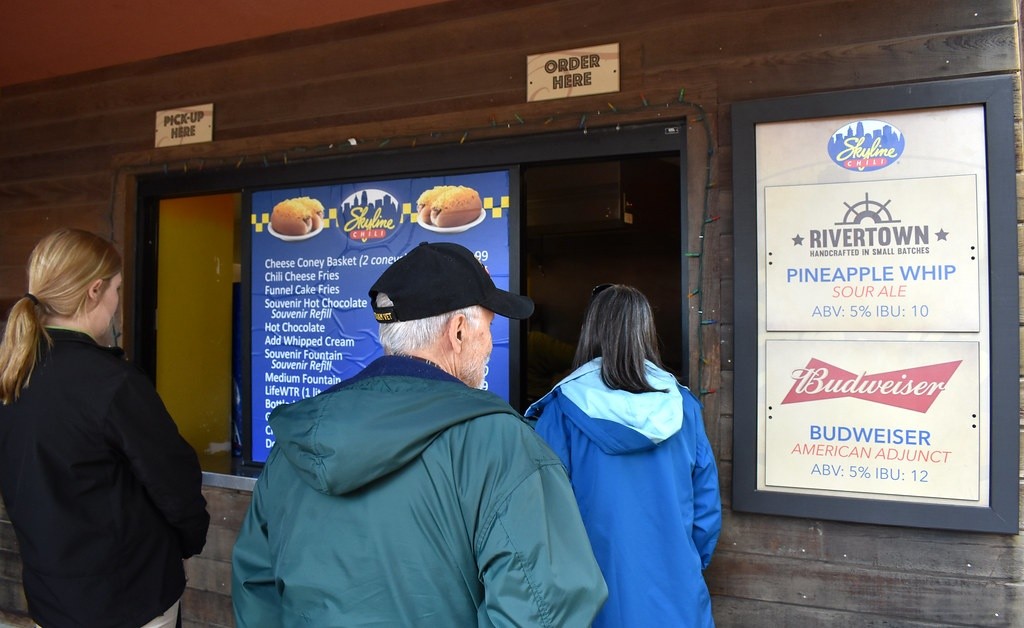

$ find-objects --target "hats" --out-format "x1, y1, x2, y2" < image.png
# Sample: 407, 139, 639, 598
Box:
367, 241, 535, 322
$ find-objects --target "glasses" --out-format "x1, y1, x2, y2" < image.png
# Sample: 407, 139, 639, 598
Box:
591, 283, 613, 295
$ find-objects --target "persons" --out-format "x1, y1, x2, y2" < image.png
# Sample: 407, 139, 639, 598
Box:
0, 229, 211, 628
520, 284, 721, 628
230, 240, 608, 628
